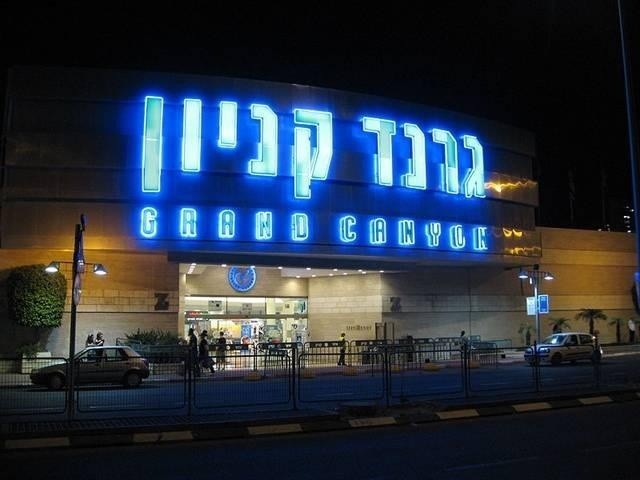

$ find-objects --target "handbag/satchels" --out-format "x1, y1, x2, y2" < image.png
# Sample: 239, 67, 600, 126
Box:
202, 357, 215, 368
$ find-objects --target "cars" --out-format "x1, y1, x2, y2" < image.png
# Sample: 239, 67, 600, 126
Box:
30, 345, 148, 389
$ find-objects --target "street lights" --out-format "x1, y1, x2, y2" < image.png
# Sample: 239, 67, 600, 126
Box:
517, 264, 554, 343
43, 214, 108, 360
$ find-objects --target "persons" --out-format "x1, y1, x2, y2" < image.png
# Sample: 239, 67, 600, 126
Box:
85, 334, 96, 361
177, 328, 226, 376
459, 331, 468, 359
95, 331, 104, 362
337, 332, 346, 365
628, 316, 635, 342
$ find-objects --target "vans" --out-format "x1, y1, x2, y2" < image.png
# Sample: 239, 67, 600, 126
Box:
525, 332, 603, 365
